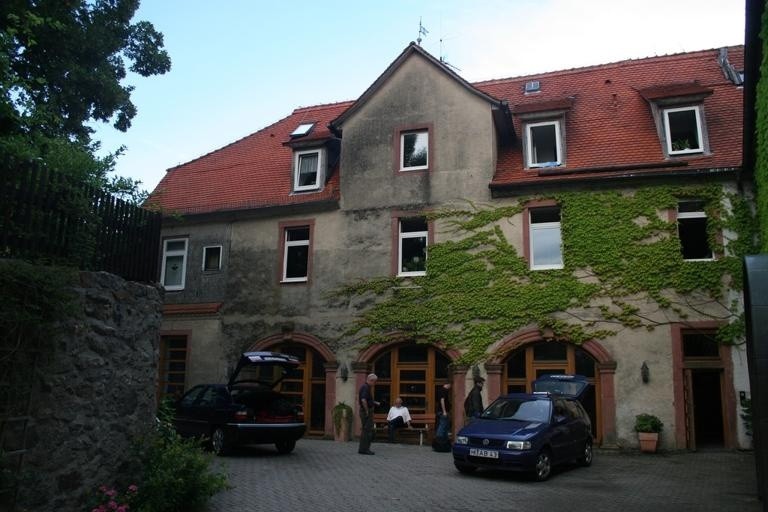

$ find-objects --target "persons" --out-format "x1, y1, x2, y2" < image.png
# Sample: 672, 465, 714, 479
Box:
387, 398, 414, 441
358, 373, 386, 455
470, 376, 486, 423
435, 379, 453, 440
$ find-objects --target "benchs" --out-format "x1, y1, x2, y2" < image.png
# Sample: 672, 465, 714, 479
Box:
371, 414, 436, 447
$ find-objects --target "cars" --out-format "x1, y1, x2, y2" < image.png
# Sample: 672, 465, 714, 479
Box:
156, 352, 308, 456
452, 372, 596, 483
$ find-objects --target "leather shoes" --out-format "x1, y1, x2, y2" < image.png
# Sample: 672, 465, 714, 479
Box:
359, 449, 374, 455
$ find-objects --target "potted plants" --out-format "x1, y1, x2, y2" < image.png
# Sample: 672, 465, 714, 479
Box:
332, 401, 352, 443
633, 413, 663, 454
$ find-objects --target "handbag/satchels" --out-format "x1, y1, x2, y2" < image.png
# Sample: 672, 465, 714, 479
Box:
464, 391, 473, 417
431, 438, 450, 452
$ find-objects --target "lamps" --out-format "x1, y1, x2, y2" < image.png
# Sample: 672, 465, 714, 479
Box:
640, 359, 650, 384
341, 361, 348, 383
